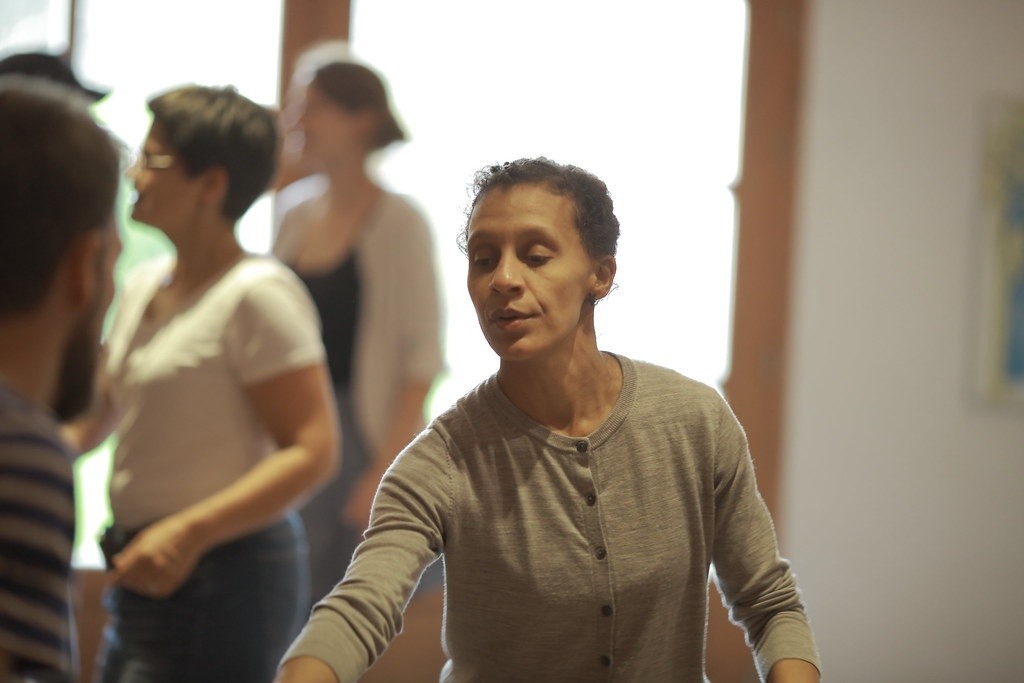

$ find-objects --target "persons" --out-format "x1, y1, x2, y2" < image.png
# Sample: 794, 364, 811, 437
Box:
273, 62, 444, 598
272, 157, 820, 683
0, 52, 123, 683
60, 87, 339, 683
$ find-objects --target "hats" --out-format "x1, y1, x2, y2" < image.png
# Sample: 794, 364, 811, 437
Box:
0, 52, 107, 110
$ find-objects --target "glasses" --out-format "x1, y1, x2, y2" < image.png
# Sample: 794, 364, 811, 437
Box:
142, 149, 194, 171
